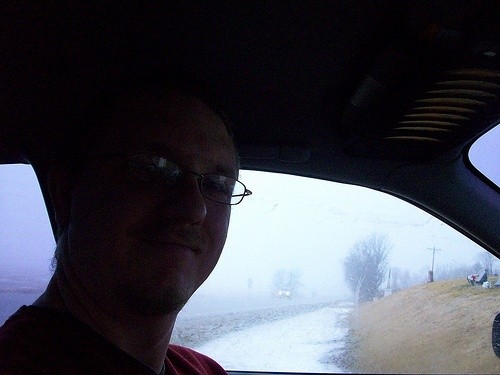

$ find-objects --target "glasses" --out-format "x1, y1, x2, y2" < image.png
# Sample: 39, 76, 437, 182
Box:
94, 153, 253, 206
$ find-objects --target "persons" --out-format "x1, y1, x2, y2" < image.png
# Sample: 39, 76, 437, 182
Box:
0, 81, 239, 375
